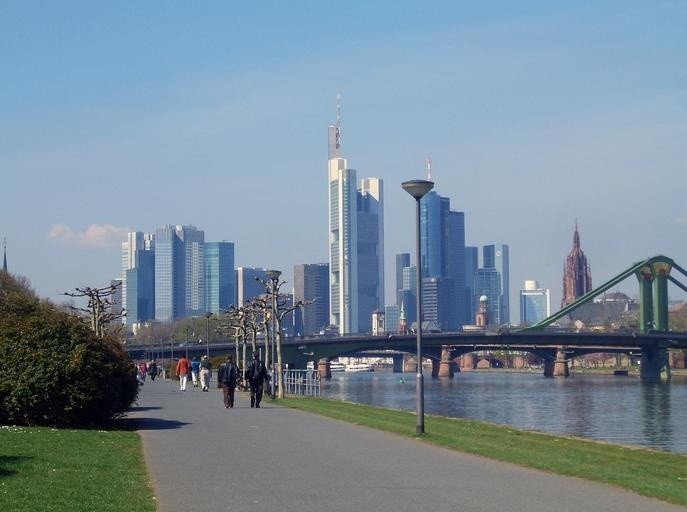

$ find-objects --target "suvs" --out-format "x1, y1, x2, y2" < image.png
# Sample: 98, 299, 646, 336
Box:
320, 325, 338, 337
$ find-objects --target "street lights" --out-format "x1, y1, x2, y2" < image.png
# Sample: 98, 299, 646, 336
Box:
204, 311, 213, 360
401, 180, 434, 434
150, 328, 188, 371
265, 270, 281, 396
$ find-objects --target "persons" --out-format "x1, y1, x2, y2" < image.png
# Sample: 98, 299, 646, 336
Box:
215, 360, 271, 396
197, 355, 212, 392
134, 359, 158, 382
190, 356, 200, 388
217, 354, 240, 409
244, 351, 268, 409
175, 355, 189, 392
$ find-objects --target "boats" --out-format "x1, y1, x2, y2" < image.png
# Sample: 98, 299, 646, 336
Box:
330, 362, 344, 370
345, 365, 374, 372
